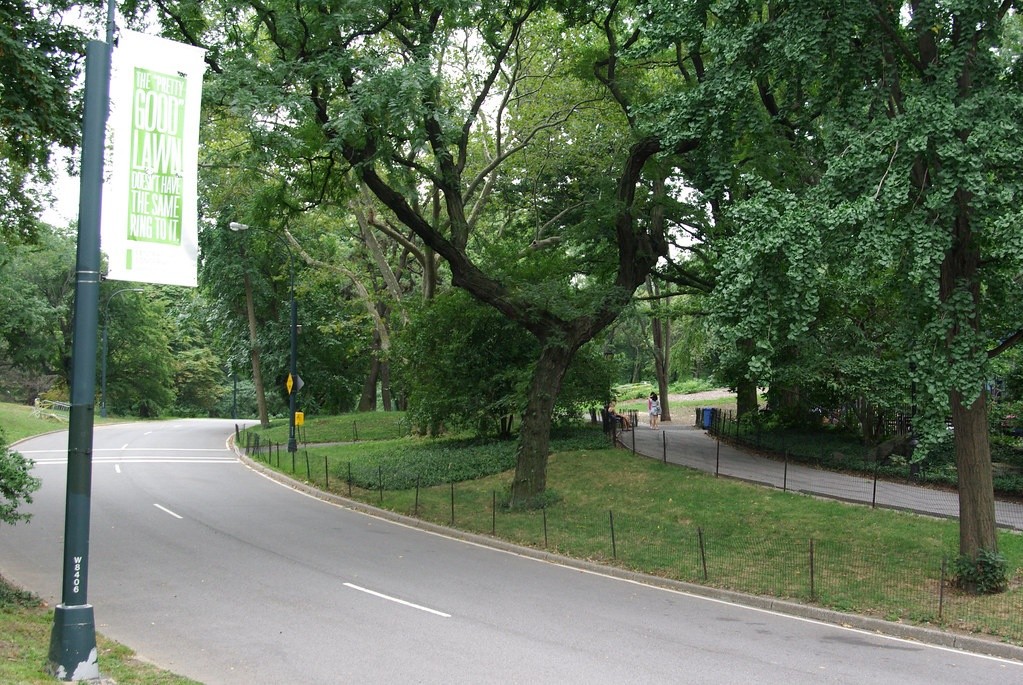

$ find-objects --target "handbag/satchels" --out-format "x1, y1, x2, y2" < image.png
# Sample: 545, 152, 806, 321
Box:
656, 406, 662, 415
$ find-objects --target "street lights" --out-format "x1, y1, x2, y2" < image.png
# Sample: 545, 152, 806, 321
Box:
230, 221, 297, 452
102, 288, 145, 418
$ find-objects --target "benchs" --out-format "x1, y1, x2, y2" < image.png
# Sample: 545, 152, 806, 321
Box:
600, 409, 623, 432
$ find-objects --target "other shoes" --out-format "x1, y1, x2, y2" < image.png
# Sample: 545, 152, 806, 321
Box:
650, 427, 653, 429
656, 428, 659, 430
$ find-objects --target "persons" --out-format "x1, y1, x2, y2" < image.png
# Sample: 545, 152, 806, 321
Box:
647, 392, 661, 429
608, 403, 633, 432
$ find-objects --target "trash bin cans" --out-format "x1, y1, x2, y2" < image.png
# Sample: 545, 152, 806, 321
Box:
702, 408, 713, 430
